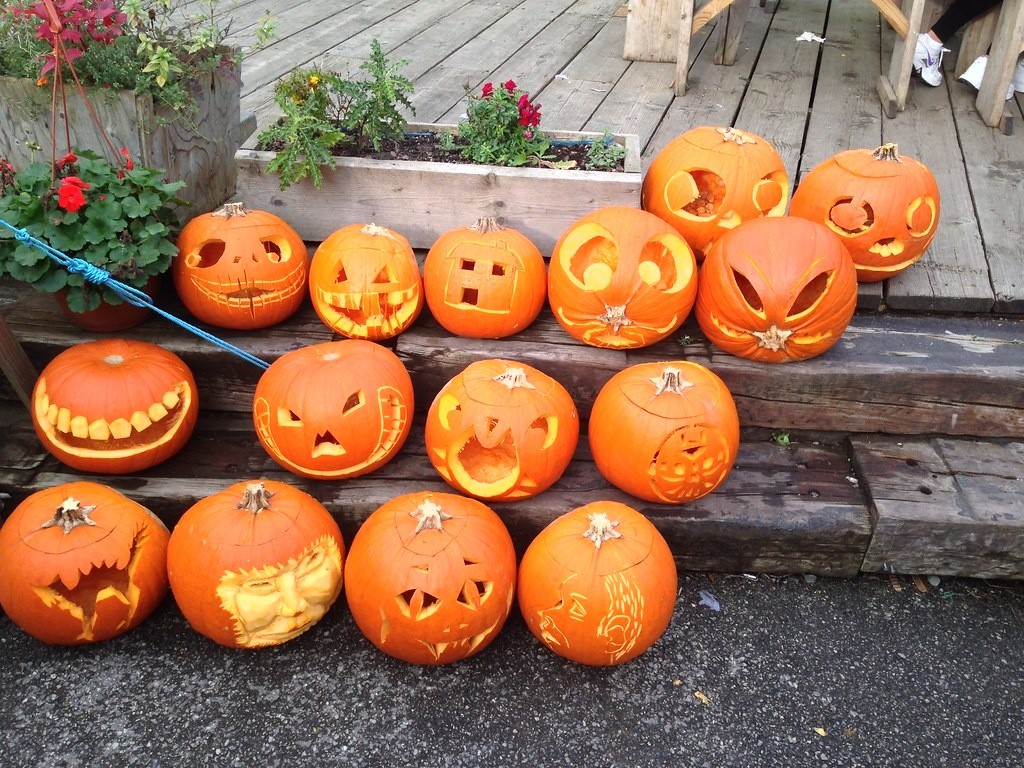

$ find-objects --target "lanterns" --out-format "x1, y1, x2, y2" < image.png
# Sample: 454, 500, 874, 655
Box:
586, 359, 741, 503
166, 479, 346, 649
308, 222, 425, 342
32, 339, 199, 476
424, 219, 546, 339
695, 216, 858, 363
254, 340, 415, 482
545, 205, 698, 352
171, 201, 308, 329
424, 359, 579, 503
789, 142, 941, 282
641, 126, 789, 261
342, 491, 518, 665
517, 500, 679, 665
1, 481, 172, 647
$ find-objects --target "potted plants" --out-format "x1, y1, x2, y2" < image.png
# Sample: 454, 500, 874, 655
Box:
234, 102, 649, 250
34, 227, 187, 329
0, 42, 246, 211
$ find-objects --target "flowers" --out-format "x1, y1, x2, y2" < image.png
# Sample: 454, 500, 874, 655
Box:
267, 52, 550, 167
0, 0, 242, 97
0, 142, 189, 305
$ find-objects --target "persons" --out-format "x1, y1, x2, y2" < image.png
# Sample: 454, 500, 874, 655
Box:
913, 0, 1015, 101
1007, 53, 1024, 93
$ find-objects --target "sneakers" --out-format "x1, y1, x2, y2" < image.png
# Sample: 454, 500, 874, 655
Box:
914, 32, 943, 87
1012, 58, 1024, 93
961, 54, 1014, 98
